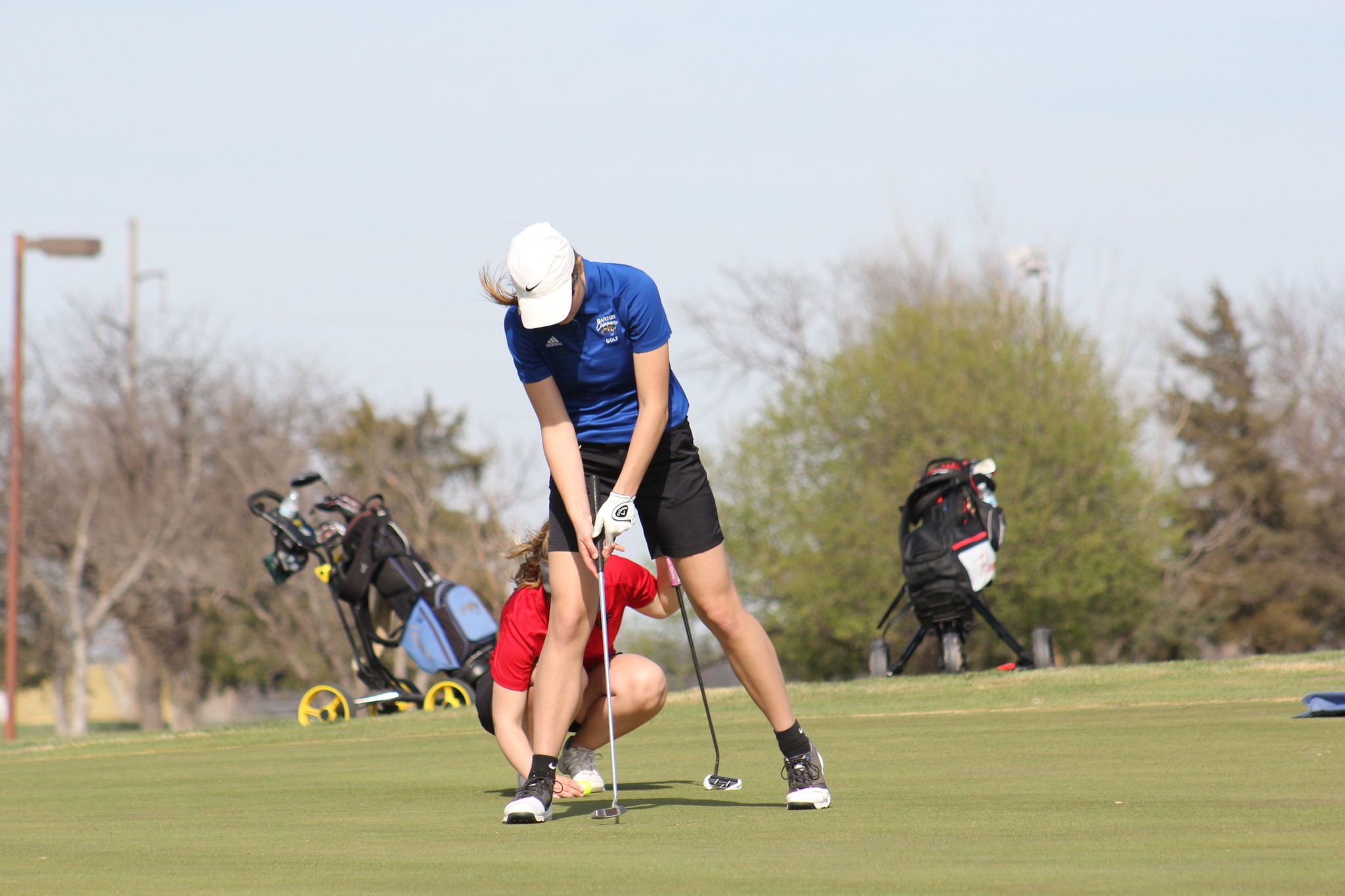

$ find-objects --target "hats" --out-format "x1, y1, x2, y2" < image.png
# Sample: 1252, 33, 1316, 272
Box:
539, 556, 551, 593
506, 222, 574, 330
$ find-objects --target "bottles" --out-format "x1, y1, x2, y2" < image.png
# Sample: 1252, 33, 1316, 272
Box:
278, 490, 301, 518
977, 482, 998, 508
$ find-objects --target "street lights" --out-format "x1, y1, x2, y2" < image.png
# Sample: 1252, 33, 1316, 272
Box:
6, 235, 102, 740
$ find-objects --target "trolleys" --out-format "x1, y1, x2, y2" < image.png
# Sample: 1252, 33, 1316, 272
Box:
867, 457, 1062, 677
247, 473, 498, 728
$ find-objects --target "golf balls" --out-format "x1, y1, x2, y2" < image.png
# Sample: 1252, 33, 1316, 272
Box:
577, 780, 592, 797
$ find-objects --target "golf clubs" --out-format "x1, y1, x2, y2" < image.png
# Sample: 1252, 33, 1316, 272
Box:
665, 556, 742, 791
587, 474, 627, 822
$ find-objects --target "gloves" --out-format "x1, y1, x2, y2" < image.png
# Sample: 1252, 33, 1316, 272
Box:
591, 491, 636, 548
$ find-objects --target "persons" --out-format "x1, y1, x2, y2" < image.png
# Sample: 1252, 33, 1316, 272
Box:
476, 519, 685, 799
478, 224, 832, 824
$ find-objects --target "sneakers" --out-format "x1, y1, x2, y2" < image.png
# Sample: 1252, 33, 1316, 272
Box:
780, 737, 831, 810
558, 736, 606, 793
502, 774, 563, 824
